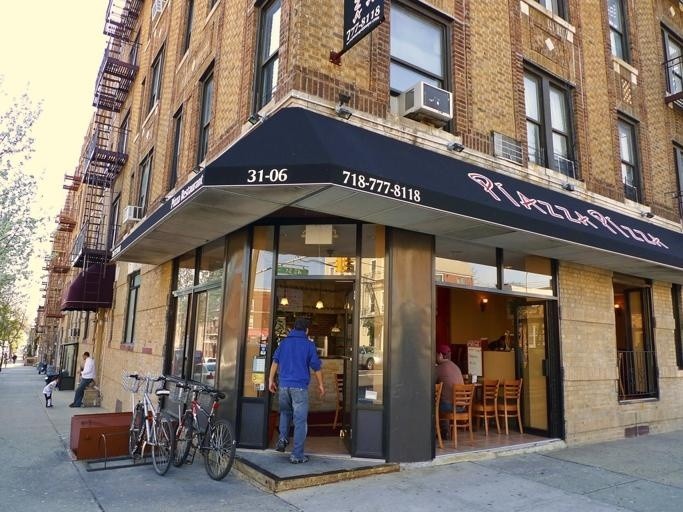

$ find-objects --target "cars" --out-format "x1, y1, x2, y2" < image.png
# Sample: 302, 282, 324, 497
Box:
173, 350, 217, 389
358, 343, 375, 370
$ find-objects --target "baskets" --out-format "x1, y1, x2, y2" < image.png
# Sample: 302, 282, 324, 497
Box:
171, 385, 200, 404
121, 370, 148, 393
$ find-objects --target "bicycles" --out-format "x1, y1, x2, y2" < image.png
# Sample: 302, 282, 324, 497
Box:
129, 374, 236, 481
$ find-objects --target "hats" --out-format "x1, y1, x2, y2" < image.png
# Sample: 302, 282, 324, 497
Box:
437, 345, 451, 353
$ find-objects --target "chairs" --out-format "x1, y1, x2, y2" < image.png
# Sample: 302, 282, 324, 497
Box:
332, 373, 345, 429
430, 378, 523, 449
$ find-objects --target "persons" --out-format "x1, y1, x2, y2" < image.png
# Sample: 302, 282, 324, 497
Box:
268, 320, 324, 465
69, 351, 95, 408
488, 335, 506, 349
436, 344, 467, 440
42, 376, 59, 408
1, 352, 17, 368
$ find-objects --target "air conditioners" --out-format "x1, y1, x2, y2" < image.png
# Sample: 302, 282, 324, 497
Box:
122, 206, 142, 224
151, 1, 162, 22
398, 80, 454, 129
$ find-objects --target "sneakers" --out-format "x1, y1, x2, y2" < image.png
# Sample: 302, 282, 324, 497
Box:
69, 404, 80, 407
437, 430, 451, 440
290, 455, 309, 463
275, 438, 287, 452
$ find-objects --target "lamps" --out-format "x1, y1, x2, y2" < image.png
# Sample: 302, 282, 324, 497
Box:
192, 165, 204, 174
447, 142, 465, 153
334, 107, 353, 120
316, 280, 324, 309
248, 113, 263, 125
280, 279, 289, 306
